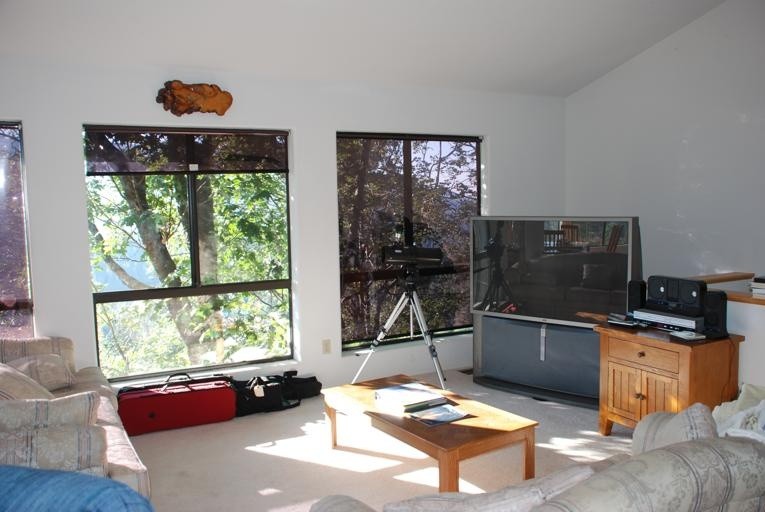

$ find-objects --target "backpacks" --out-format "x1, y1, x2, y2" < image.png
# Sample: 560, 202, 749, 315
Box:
235, 371, 300, 418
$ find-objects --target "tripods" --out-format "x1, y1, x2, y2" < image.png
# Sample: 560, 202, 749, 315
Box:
351, 268, 446, 391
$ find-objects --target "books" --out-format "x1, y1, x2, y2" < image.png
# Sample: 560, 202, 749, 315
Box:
749, 276, 765, 302
375, 381, 471, 429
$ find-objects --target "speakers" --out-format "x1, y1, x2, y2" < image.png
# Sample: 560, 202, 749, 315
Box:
628, 280, 646, 314
704, 290, 729, 339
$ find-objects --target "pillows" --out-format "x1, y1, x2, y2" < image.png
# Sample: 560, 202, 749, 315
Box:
0, 352, 75, 389
0, 337, 73, 362
580, 262, 612, 289
0, 363, 56, 402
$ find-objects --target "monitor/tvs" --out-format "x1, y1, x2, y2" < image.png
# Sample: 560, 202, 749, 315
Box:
469, 216, 643, 330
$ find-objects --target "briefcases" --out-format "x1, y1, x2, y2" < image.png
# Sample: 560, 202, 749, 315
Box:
117, 374, 236, 437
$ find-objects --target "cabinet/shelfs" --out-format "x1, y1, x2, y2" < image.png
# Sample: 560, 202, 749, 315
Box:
472, 314, 599, 408
593, 326, 745, 440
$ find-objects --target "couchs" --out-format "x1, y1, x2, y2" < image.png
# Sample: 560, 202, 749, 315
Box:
311, 386, 765, 512
509, 251, 628, 314
0, 334, 151, 499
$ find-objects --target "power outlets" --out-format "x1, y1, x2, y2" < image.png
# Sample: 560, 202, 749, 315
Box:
320, 340, 331, 354
